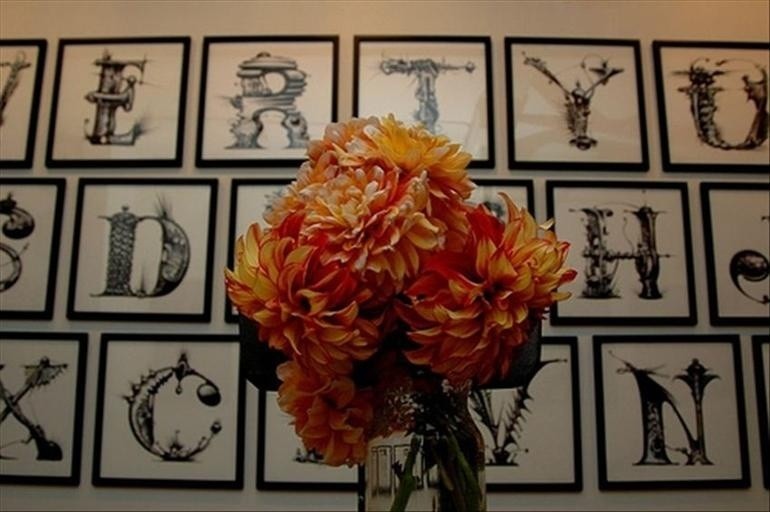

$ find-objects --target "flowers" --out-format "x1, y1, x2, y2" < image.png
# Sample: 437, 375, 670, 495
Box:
223, 109, 578, 512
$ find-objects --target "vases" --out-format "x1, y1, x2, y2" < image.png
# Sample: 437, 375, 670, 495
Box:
356, 430, 491, 512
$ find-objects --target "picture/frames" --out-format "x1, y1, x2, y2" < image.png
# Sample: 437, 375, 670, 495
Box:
590, 333, 752, 490
465, 336, 583, 490
45, 35, 191, 169
0, 329, 91, 489
351, 36, 497, 171
255, 388, 358, 490
468, 177, 540, 333
91, 333, 247, 489
546, 179, 699, 328
751, 334, 769, 490
67, 176, 218, 325
701, 182, 770, 327
653, 40, 769, 174
1, 38, 47, 170
2, 175, 67, 322
225, 176, 300, 324
195, 37, 339, 169
503, 37, 651, 173
